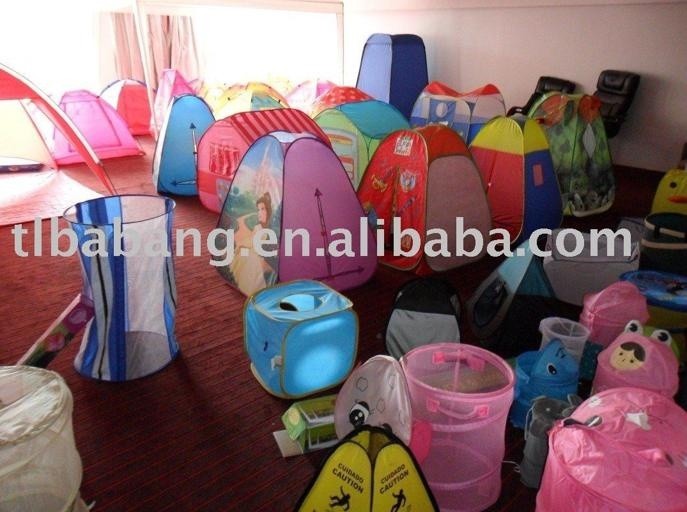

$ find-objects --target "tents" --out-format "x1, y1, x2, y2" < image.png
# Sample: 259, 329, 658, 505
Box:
149, 69, 196, 142
311, 101, 408, 197
211, 81, 288, 114
195, 109, 331, 214
214, 92, 291, 123
305, 88, 374, 119
411, 81, 506, 149
212, 130, 379, 298
34, 90, 144, 166
98, 78, 157, 136
283, 78, 336, 110
466, 114, 564, 248
528, 94, 614, 216
0, 65, 116, 230
151, 95, 215, 197
358, 123, 493, 278
354, 34, 429, 121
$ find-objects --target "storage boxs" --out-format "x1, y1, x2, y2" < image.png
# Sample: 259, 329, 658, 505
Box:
272, 347, 516, 458
